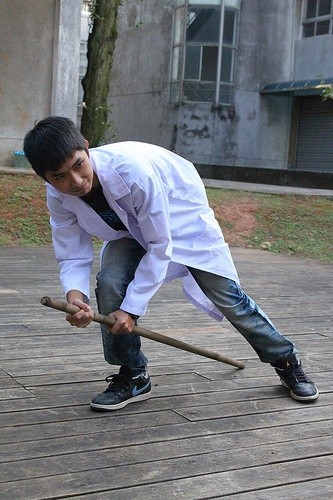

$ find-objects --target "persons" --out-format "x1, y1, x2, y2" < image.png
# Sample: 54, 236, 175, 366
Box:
23, 114, 321, 411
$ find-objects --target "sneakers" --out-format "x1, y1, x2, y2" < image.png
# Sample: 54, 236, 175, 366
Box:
90, 364, 151, 411
273, 353, 319, 402
